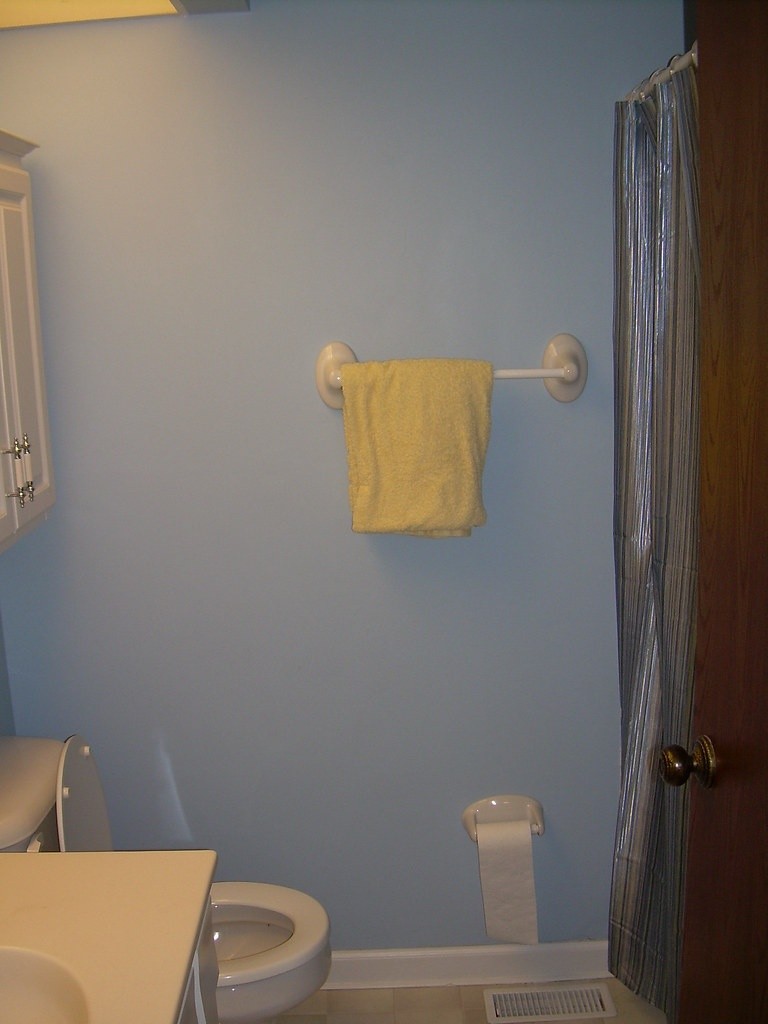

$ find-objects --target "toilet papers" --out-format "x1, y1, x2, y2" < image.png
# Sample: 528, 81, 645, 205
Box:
475, 819, 533, 848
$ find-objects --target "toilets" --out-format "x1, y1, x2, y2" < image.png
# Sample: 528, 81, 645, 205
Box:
0, 733, 334, 1024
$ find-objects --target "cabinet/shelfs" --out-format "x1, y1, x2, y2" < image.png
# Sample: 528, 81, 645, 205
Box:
177, 887, 218, 1024
0, 130, 56, 553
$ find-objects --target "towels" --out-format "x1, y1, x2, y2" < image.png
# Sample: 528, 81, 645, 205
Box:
337, 357, 493, 539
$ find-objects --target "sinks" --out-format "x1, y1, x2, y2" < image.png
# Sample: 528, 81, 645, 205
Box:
0, 946, 88, 1024
